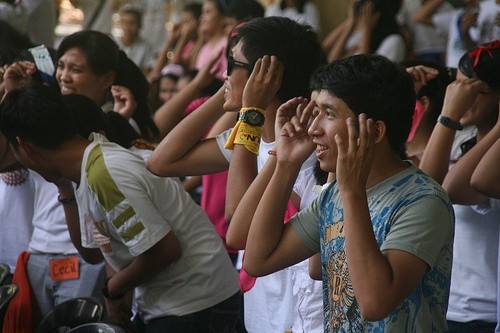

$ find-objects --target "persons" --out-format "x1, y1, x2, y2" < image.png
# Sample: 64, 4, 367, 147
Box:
0, 0, 500, 333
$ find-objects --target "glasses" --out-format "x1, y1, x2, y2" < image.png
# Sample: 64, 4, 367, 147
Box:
227, 55, 253, 76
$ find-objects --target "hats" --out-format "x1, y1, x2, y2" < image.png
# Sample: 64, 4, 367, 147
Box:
459, 39, 500, 82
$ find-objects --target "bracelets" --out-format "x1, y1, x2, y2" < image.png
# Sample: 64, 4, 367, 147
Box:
58, 194, 74, 203
437, 115, 464, 130
268, 151, 276, 155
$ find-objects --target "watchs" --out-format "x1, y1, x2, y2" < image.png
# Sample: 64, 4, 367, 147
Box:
237, 109, 265, 126
